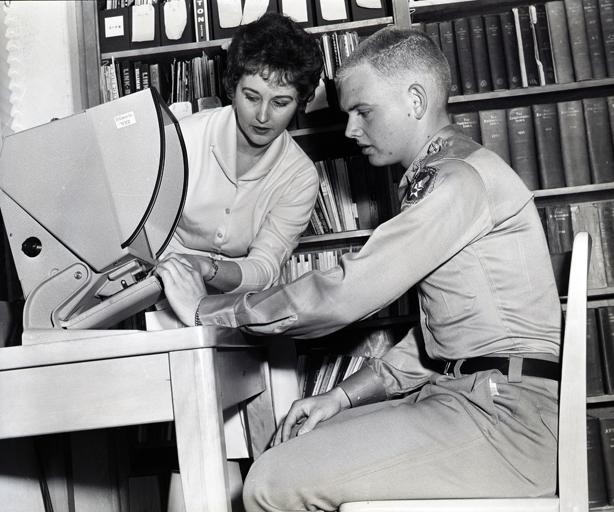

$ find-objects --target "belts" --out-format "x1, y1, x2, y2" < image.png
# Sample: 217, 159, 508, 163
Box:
441, 356, 561, 379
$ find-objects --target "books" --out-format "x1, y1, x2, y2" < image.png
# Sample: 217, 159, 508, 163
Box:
559, 305, 614, 397
96, 0, 405, 238
408, 2, 613, 97
280, 241, 420, 398
535, 198, 614, 297
447, 95, 614, 190
586, 414, 614, 509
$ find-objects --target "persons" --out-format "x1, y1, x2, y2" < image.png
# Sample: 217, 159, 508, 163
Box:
136, 12, 324, 512
145, 27, 565, 512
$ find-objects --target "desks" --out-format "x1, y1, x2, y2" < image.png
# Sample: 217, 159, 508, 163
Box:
0, 326, 277, 512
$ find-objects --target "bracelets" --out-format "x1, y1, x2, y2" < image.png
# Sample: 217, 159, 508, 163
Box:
195, 296, 207, 326
204, 256, 218, 282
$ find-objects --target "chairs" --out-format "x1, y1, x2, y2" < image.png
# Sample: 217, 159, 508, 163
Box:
338, 231, 592, 512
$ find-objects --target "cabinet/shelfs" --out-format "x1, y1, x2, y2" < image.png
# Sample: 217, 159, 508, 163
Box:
69, 0, 613, 512
